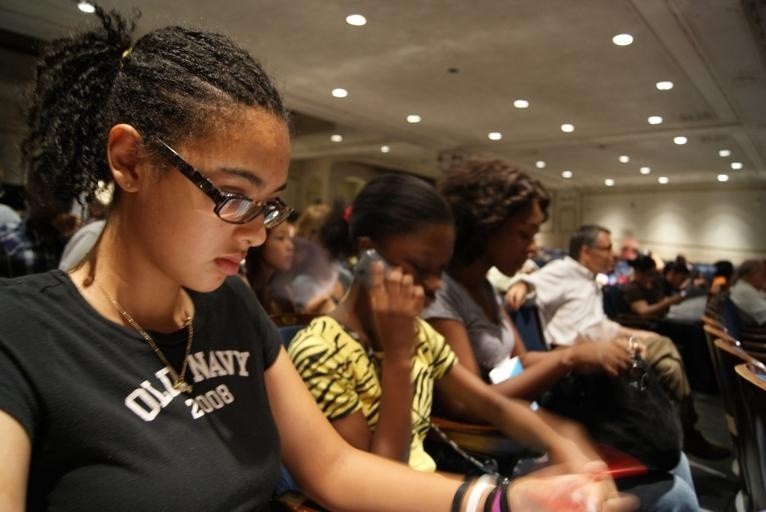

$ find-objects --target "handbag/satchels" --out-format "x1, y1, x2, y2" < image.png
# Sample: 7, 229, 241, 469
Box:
577, 357, 680, 477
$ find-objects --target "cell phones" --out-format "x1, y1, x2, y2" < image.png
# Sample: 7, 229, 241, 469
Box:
350, 249, 392, 293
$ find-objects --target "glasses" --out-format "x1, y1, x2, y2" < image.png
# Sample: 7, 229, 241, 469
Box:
146, 135, 294, 230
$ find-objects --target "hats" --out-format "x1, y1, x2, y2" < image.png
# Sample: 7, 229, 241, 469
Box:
626, 255, 655, 272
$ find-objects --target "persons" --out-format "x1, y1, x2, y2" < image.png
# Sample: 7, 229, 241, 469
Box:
0, 157, 765, 511
0, 2, 643, 510
